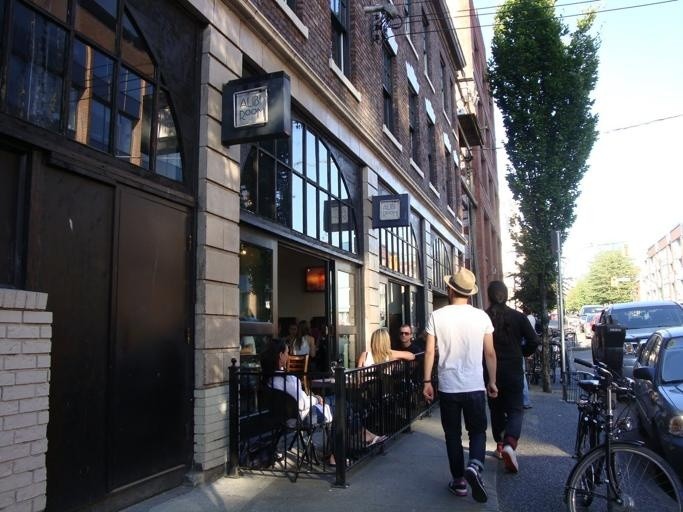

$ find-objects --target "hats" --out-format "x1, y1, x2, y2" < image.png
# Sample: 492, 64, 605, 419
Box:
442, 267, 478, 296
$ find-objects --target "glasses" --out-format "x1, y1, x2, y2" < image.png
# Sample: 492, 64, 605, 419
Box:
399, 332, 409, 336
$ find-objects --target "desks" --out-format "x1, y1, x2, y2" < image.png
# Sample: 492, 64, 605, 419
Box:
312, 375, 378, 425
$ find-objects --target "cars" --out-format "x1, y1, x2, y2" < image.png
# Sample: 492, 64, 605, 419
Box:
633, 325, 683, 461
565, 300, 681, 371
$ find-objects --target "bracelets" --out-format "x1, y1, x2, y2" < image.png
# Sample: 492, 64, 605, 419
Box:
423, 380, 431, 383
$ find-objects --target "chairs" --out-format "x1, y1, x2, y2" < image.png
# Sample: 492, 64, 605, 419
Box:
262, 387, 330, 483
286, 354, 310, 395
360, 374, 411, 454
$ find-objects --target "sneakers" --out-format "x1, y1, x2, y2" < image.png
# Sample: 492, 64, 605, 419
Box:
524, 402, 534, 409
492, 449, 503, 459
463, 463, 487, 503
500, 445, 518, 473
447, 479, 467, 497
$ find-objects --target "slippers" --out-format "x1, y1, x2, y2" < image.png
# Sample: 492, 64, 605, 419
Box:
365, 435, 388, 448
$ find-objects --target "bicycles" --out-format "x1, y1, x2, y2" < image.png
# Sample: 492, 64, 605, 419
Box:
564, 357, 683, 510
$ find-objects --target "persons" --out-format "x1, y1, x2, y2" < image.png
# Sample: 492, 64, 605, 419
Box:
286, 323, 297, 351
293, 320, 317, 379
259, 339, 389, 468
391, 325, 427, 384
356, 328, 415, 401
523, 305, 537, 331
521, 338, 533, 411
421, 268, 500, 504
482, 281, 539, 473
